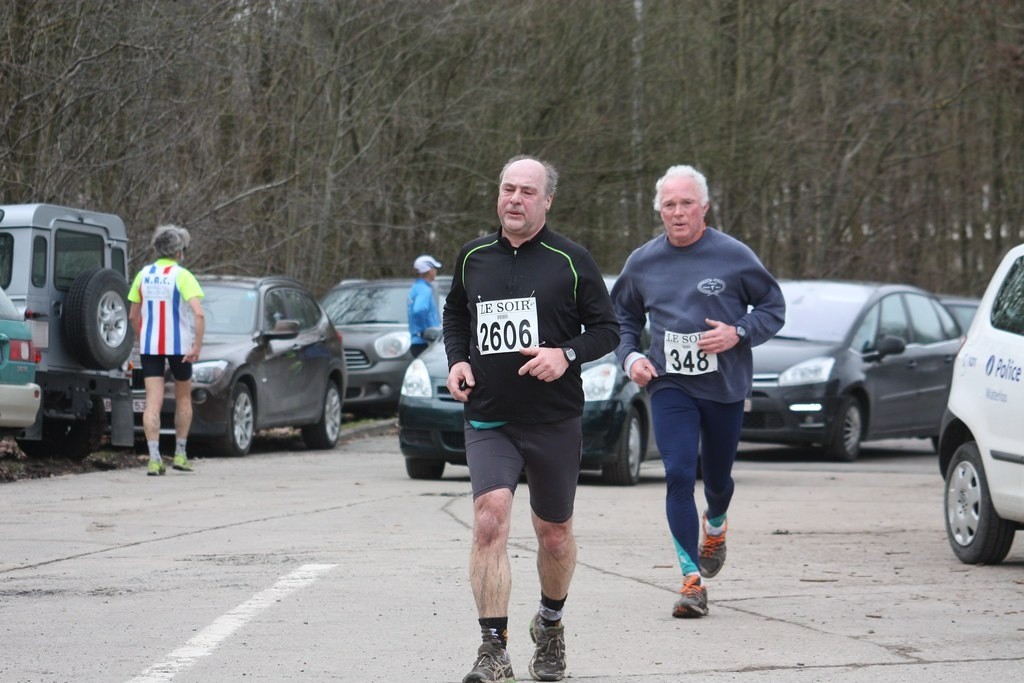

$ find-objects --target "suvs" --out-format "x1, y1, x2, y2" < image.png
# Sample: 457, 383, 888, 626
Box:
101, 272, 347, 456
0, 202, 147, 468
316, 276, 454, 417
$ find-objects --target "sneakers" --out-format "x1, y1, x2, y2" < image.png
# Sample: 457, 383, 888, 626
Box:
462, 629, 515, 683
147, 459, 166, 475
528, 614, 566, 681
172, 454, 193, 472
673, 575, 708, 619
697, 510, 728, 578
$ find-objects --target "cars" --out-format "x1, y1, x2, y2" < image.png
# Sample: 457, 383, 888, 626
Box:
397, 274, 704, 487
736, 279, 984, 460
0, 285, 41, 438
936, 242, 1024, 565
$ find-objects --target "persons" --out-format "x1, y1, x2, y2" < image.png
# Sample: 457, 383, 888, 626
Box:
442, 154, 621, 683
408, 255, 443, 359
128, 224, 205, 477
610, 165, 786, 618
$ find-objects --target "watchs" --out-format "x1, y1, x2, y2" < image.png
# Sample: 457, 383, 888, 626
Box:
735, 325, 745, 340
562, 347, 576, 366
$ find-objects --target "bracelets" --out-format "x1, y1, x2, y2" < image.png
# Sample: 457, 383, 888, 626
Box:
625, 353, 645, 380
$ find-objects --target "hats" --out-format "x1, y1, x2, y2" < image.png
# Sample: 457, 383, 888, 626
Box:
153, 225, 190, 256
413, 255, 442, 274
